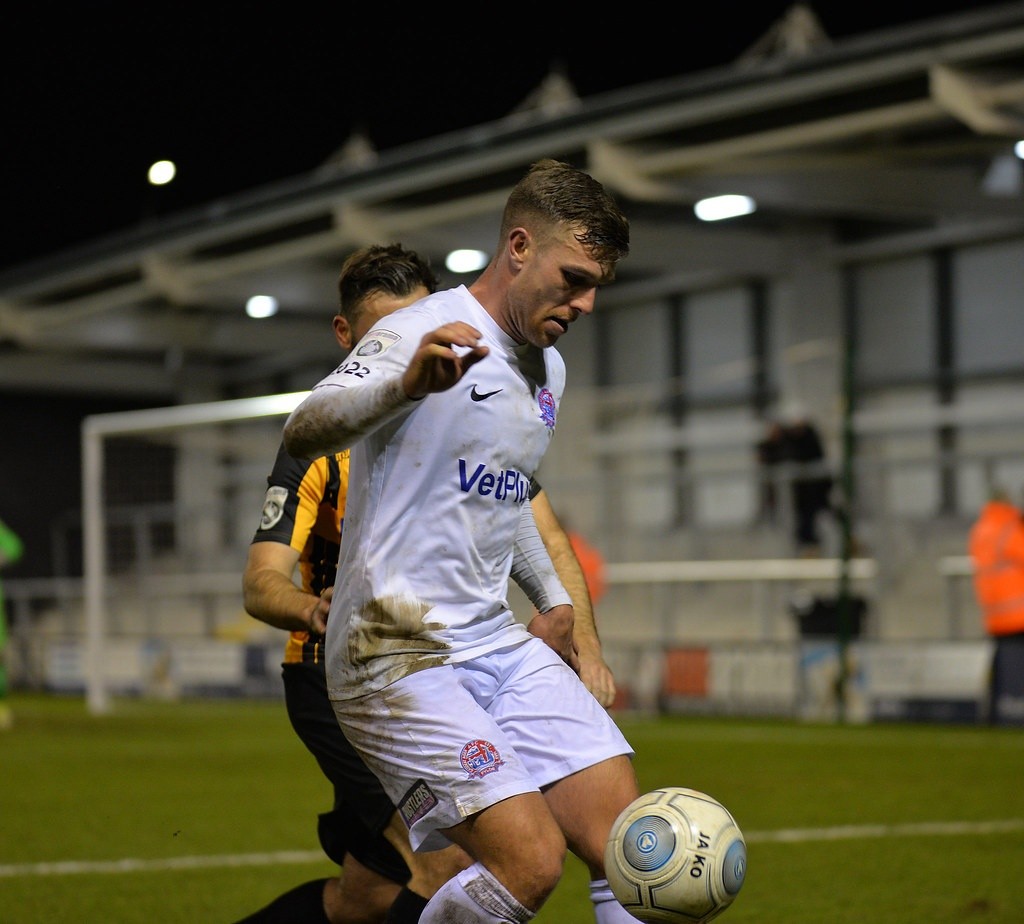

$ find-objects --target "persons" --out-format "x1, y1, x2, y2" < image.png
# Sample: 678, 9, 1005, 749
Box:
968, 485, 1024, 734
273, 159, 676, 922
228, 238, 619, 922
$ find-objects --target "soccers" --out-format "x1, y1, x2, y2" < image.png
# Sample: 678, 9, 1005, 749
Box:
603, 787, 748, 924
